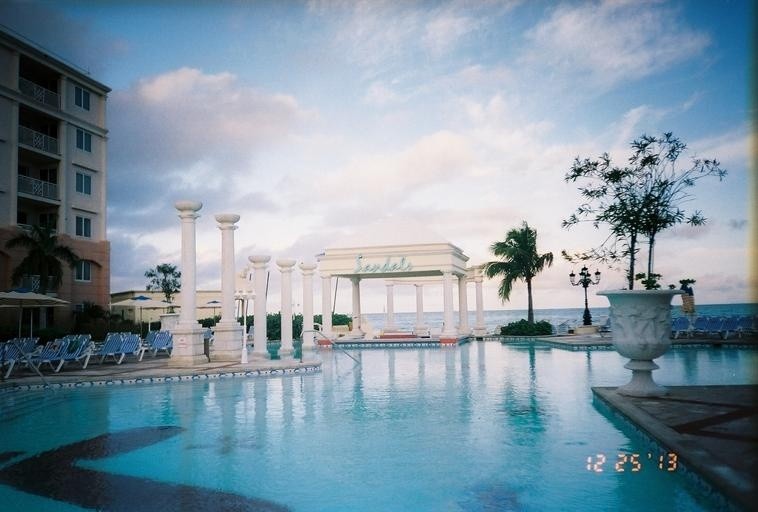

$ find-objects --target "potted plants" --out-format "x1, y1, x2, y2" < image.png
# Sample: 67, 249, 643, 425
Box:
560, 133, 727, 399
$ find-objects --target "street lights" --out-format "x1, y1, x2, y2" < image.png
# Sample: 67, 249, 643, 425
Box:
234, 286, 257, 365
567, 264, 601, 324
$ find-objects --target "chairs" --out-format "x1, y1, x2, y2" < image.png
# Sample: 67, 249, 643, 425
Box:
0, 329, 175, 378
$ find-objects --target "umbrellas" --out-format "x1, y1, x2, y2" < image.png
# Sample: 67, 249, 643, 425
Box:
0, 292, 73, 342
138, 298, 181, 331
196, 300, 222, 326
0, 290, 62, 339
106, 295, 169, 338
